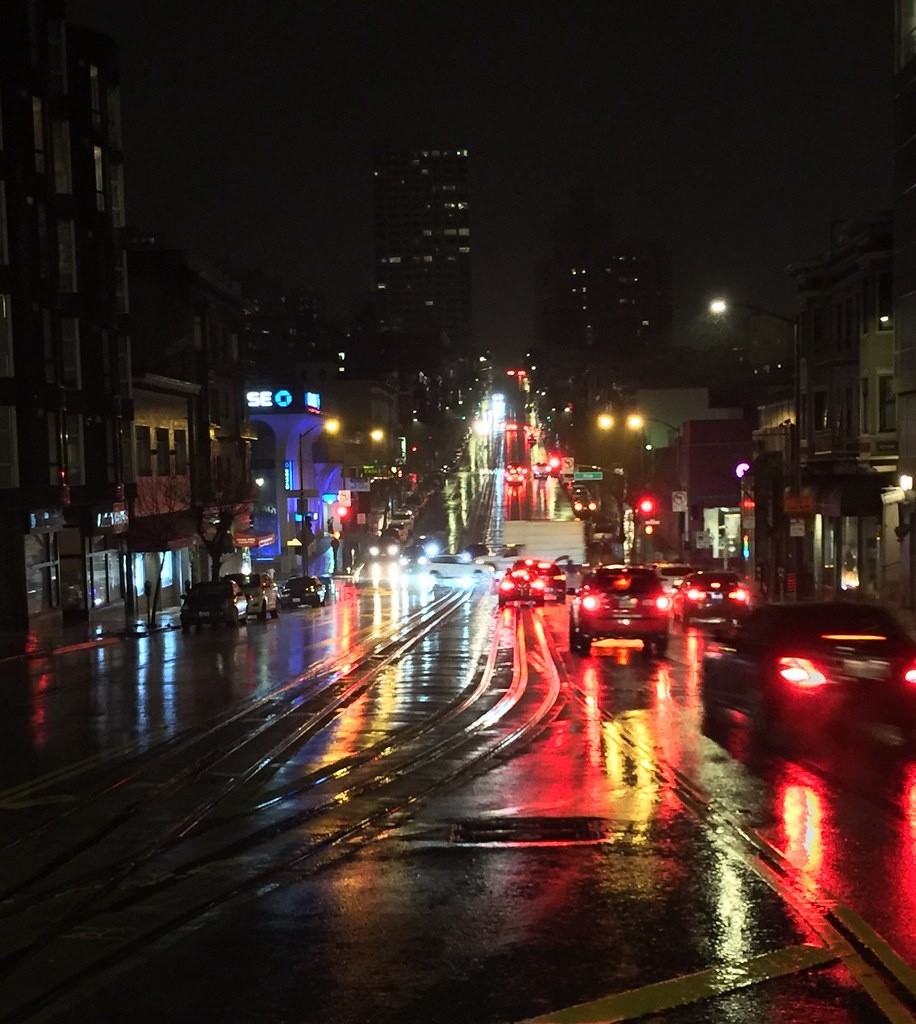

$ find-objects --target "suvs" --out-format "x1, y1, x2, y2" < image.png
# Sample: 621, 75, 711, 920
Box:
563, 564, 676, 659
219, 572, 279, 622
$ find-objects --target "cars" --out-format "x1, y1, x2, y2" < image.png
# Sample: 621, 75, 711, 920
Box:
673, 570, 756, 626
702, 598, 916, 754
180, 579, 249, 631
354, 369, 692, 608
279, 575, 327, 608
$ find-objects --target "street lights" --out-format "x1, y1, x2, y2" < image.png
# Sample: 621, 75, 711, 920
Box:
711, 300, 801, 490
628, 415, 680, 492
342, 425, 385, 489
297, 419, 338, 582
596, 412, 646, 481
